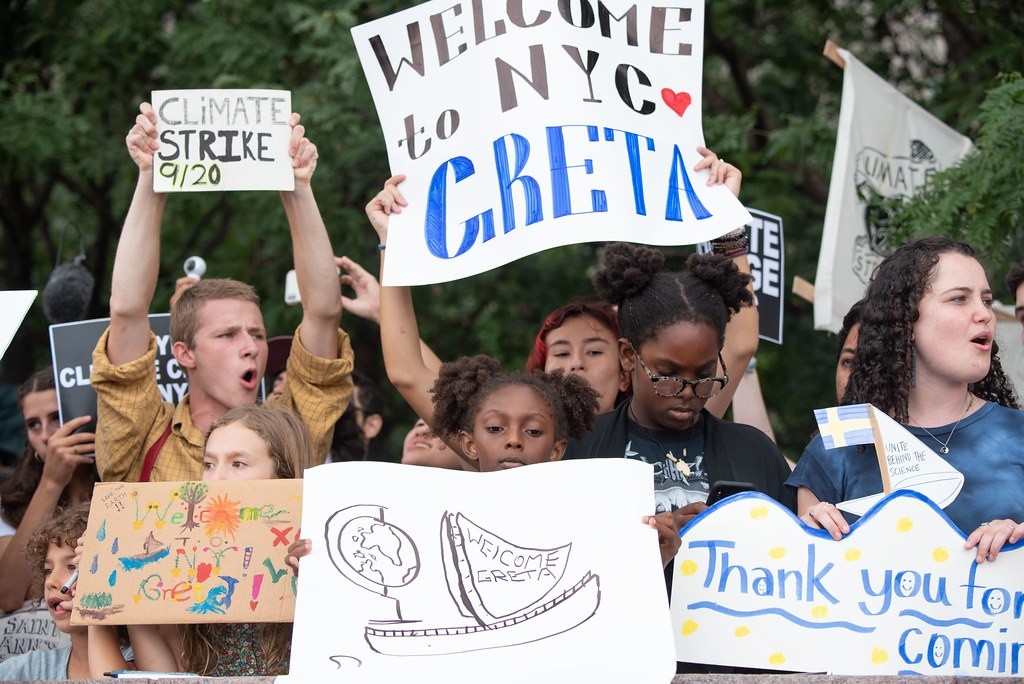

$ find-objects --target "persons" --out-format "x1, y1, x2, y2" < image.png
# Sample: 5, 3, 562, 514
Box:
334, 145, 792, 608
89, 102, 355, 481
0, 337, 389, 684
783, 237, 1024, 564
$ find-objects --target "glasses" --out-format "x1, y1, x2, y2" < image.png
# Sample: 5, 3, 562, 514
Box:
628, 341, 729, 399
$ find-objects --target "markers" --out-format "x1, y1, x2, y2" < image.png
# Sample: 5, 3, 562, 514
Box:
61, 569, 79, 594
103, 670, 197, 678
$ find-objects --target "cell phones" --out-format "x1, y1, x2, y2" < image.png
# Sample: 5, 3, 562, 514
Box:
706, 481, 754, 506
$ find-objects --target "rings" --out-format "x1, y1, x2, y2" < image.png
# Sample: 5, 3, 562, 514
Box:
981, 522, 991, 530
1007, 524, 1015, 532
809, 510, 817, 519
824, 502, 835, 510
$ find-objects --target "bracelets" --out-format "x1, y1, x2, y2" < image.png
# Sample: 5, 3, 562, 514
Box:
378, 243, 386, 251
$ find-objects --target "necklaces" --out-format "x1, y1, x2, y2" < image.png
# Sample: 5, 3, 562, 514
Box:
909, 391, 974, 455
